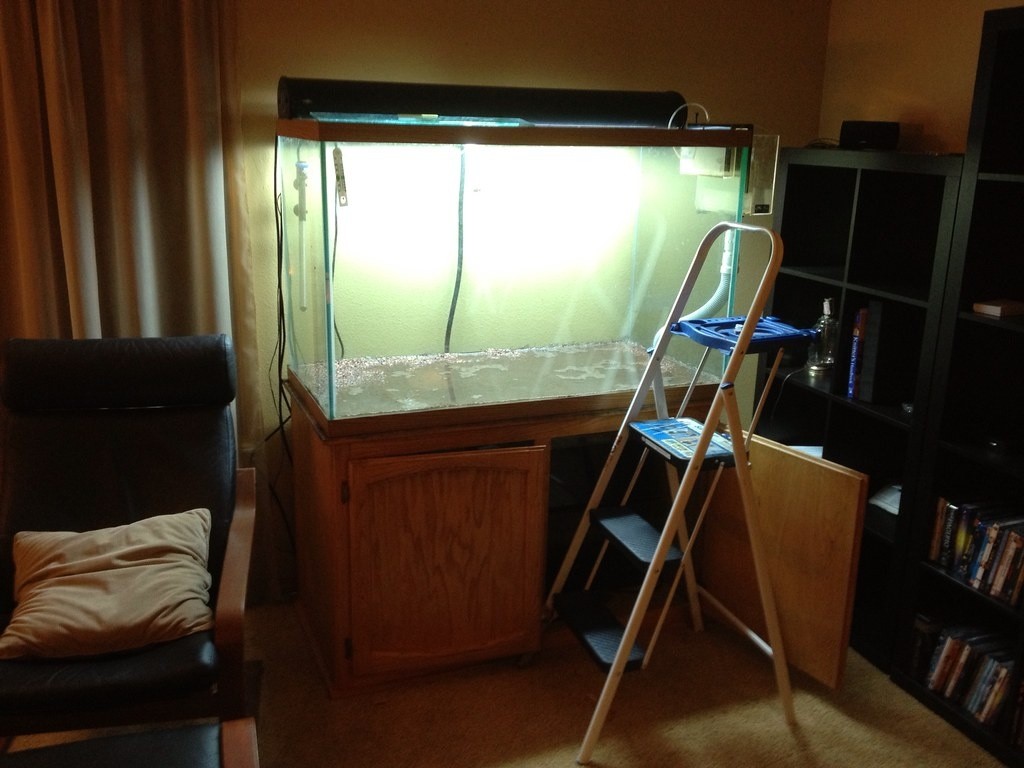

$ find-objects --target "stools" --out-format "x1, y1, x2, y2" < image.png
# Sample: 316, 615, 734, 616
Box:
1, 717, 259, 768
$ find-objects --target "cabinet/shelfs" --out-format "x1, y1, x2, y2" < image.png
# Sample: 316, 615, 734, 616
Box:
751, 143, 965, 662
279, 335, 867, 691
885, 7, 1024, 766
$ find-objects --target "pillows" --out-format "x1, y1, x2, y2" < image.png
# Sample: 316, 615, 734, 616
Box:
1, 506, 210, 657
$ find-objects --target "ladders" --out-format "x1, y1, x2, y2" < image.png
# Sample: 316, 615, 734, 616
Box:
546, 218, 806, 765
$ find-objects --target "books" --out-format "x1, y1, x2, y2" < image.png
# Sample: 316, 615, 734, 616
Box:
905, 494, 1024, 725
847, 306, 883, 403
971, 298, 1023, 317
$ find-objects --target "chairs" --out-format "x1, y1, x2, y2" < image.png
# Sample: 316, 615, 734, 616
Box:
1, 334, 256, 747
